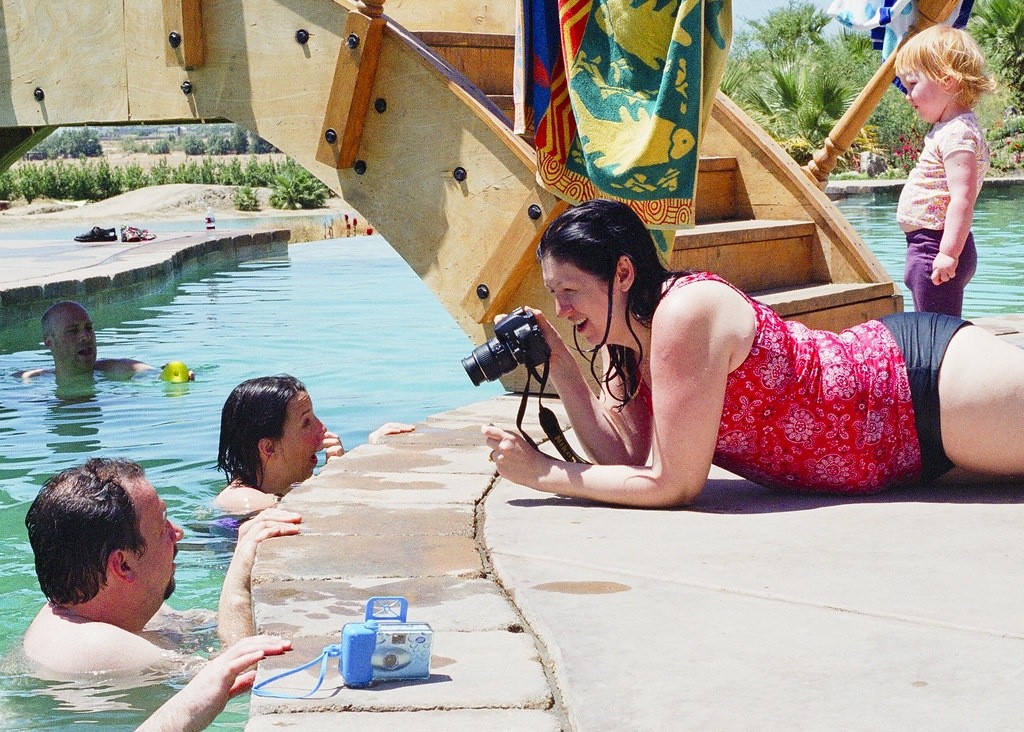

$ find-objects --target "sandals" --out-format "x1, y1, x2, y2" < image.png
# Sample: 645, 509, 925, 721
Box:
74, 226, 117, 242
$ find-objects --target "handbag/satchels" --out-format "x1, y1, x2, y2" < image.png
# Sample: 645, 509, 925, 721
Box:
121, 225, 157, 242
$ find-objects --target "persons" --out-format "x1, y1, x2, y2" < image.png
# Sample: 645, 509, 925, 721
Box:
12, 301, 194, 380
895, 26, 990, 317
482, 199, 1024, 510
214, 377, 416, 514
23, 461, 302, 674
135, 636, 291, 732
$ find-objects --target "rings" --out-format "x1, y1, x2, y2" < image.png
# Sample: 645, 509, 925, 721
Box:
488, 450, 496, 463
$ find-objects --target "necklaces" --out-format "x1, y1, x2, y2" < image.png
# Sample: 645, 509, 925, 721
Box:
625, 327, 651, 399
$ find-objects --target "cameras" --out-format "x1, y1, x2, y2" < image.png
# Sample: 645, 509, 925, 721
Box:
337, 596, 433, 688
461, 307, 551, 387
